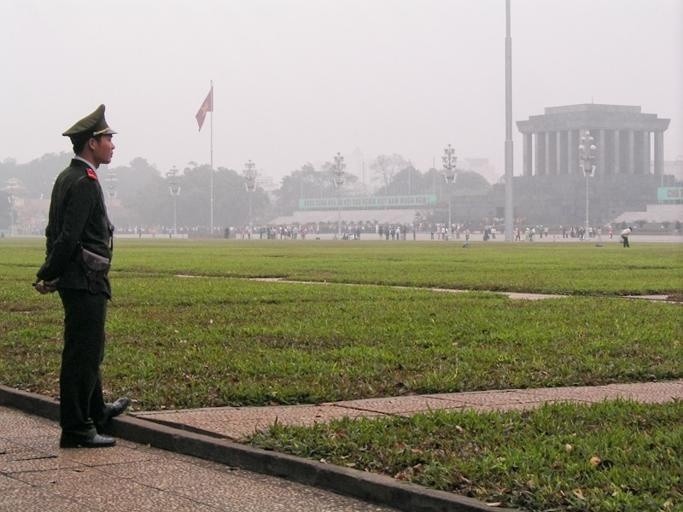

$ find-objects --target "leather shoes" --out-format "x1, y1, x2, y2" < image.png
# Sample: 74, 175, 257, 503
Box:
58, 396, 130, 448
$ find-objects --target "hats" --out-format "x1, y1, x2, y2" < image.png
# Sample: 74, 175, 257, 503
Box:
62, 103, 118, 147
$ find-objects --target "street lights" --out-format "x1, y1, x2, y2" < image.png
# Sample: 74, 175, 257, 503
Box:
440, 144, 457, 233
243, 158, 257, 232
331, 151, 346, 236
166, 166, 181, 235
579, 130, 597, 238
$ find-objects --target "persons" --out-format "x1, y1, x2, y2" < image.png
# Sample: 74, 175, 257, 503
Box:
32, 101, 132, 447
132, 219, 633, 252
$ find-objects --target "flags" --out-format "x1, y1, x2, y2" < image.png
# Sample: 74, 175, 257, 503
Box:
195, 88, 214, 130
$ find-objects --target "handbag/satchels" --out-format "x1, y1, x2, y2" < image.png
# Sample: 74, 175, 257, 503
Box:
74, 242, 111, 284
